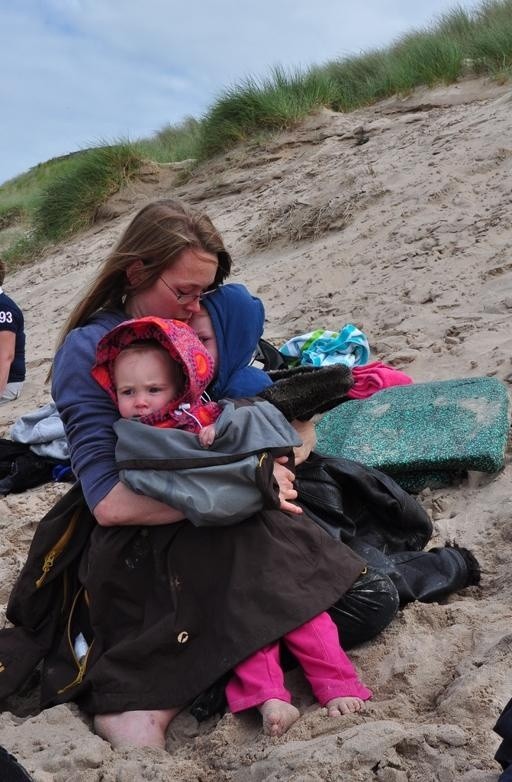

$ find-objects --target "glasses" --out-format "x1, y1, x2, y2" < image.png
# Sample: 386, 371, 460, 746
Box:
159, 276, 216, 305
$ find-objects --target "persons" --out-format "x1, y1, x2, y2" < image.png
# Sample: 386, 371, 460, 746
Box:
0, 259, 25, 406
44, 199, 399, 749
90, 317, 373, 736
189, 284, 273, 399
